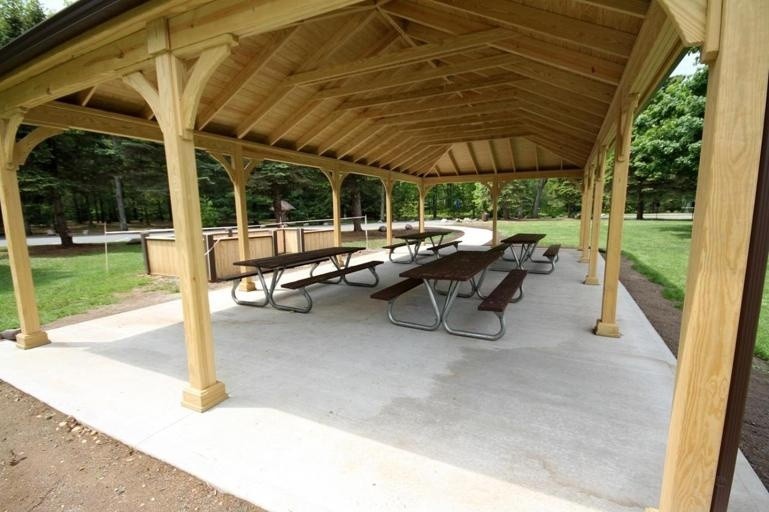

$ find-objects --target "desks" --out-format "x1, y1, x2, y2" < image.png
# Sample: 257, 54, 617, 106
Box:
499, 232, 546, 273
395, 231, 451, 264
398, 250, 504, 338
233, 248, 367, 309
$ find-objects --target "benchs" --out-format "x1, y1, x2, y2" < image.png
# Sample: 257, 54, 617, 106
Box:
281, 261, 385, 313
426, 241, 462, 260
370, 279, 428, 323
217, 256, 330, 304
488, 243, 512, 263
382, 240, 424, 263
543, 244, 562, 274
478, 268, 529, 340
